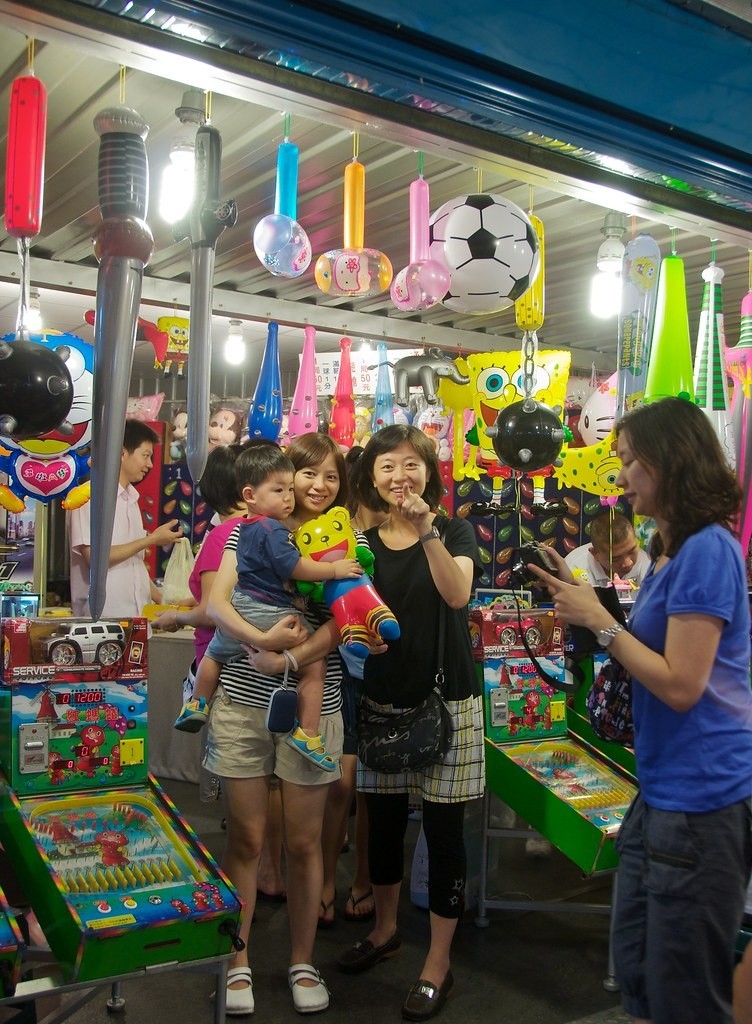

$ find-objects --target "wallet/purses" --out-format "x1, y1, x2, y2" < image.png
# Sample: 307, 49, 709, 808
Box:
267, 652, 298, 734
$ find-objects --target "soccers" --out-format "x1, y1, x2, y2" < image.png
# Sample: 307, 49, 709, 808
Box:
429, 192, 540, 316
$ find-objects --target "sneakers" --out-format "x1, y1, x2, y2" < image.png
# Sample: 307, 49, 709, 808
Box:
174, 698, 209, 733
284, 720, 336, 772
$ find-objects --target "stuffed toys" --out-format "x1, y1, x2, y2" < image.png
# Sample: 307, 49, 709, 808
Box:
168, 404, 450, 461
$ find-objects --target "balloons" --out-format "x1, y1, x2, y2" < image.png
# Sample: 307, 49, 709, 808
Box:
437, 480, 625, 587
0, 329, 95, 513
252, 145, 752, 514
292, 506, 401, 658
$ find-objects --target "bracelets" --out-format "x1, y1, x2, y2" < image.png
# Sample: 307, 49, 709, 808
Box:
173, 611, 184, 631
282, 649, 298, 672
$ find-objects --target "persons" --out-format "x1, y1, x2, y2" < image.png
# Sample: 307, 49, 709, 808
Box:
526, 396, 752, 1024
69, 418, 182, 617
563, 509, 651, 600
147, 425, 485, 1020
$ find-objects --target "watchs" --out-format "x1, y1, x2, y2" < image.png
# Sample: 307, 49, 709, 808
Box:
597, 624, 624, 648
418, 525, 441, 542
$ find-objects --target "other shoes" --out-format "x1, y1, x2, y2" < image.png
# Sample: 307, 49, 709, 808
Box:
402, 966, 454, 1020
226, 966, 254, 1014
338, 930, 401, 968
288, 964, 328, 1013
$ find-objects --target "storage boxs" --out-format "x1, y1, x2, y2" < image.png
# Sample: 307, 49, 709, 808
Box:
0, 615, 149, 685
466, 608, 565, 659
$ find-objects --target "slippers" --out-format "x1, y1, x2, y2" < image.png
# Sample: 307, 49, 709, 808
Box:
317, 888, 338, 928
344, 887, 375, 921
257, 886, 287, 903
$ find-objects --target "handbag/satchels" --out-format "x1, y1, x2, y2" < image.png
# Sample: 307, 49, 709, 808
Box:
587, 646, 638, 744
162, 537, 200, 606
354, 685, 453, 775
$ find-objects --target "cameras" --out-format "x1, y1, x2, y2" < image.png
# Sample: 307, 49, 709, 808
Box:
512, 542, 559, 582
